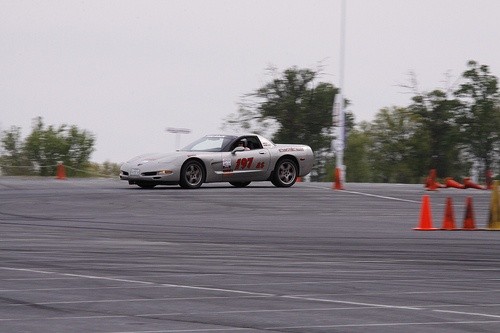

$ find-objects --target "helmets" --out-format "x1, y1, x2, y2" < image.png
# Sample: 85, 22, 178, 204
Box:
239, 139, 248, 146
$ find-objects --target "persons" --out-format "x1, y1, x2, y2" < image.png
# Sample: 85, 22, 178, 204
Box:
238, 139, 250, 151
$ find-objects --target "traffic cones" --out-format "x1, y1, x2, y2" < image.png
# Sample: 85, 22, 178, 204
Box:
482, 180, 499, 232
441, 196, 459, 231
411, 194, 442, 231
332, 167, 344, 190
422, 168, 497, 192
54, 163, 68, 181
461, 195, 481, 231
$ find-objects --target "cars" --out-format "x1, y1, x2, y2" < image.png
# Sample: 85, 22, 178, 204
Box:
119, 133, 315, 190
